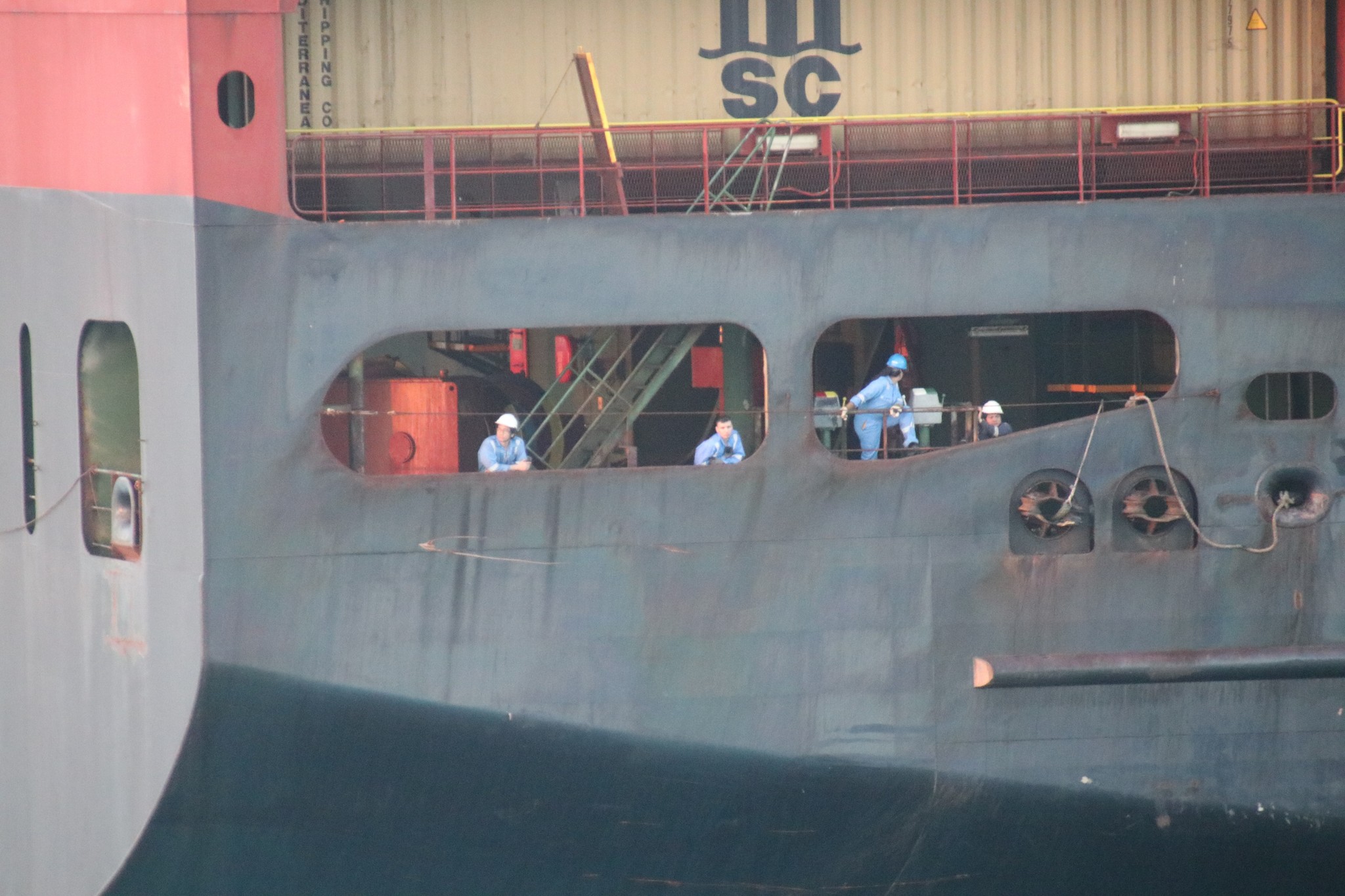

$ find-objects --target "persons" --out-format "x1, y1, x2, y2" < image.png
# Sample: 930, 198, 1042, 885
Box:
959, 400, 1013, 445
477, 412, 532, 472
693, 413, 745, 465
837, 353, 930, 463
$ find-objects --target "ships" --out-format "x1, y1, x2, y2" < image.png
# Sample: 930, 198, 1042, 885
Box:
0, 1, 1343, 896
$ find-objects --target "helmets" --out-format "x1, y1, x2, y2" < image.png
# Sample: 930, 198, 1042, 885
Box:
495, 413, 518, 429
887, 354, 907, 370
982, 400, 1004, 414
